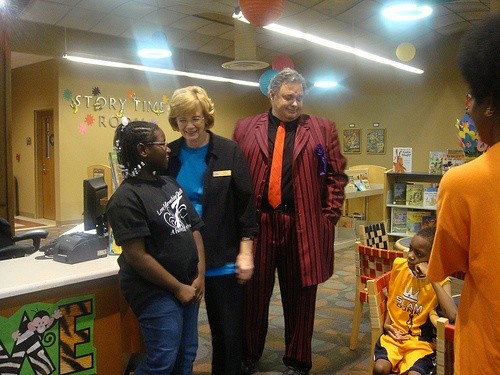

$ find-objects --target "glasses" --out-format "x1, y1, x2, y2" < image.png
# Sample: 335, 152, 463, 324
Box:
144, 142, 167, 149
464, 92, 475, 115
175, 115, 205, 125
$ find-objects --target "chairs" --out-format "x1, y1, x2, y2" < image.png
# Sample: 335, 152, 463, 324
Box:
350, 220, 455, 375
0, 217, 48, 261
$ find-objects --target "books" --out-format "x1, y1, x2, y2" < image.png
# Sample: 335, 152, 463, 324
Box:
390, 207, 436, 235
392, 180, 440, 208
338, 173, 371, 191
427, 149, 481, 175
391, 146, 413, 174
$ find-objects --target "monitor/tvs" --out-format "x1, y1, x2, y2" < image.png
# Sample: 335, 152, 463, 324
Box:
84, 176, 108, 236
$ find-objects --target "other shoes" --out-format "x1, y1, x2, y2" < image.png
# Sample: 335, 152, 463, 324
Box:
240, 364, 255, 375
282, 366, 305, 375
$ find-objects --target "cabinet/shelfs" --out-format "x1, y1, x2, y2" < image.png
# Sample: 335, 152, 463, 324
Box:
383, 169, 443, 237
333, 165, 387, 251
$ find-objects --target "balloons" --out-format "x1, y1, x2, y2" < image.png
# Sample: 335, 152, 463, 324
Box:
259, 55, 294, 98
238, 0, 285, 28
395, 43, 417, 62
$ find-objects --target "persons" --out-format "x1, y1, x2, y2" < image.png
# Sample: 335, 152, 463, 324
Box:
426, 10, 500, 375
459, 91, 483, 158
232, 68, 349, 375
106, 121, 205, 375
372, 226, 458, 375
154, 86, 256, 375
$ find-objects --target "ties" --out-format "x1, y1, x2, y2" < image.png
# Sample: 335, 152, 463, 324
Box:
268, 122, 285, 209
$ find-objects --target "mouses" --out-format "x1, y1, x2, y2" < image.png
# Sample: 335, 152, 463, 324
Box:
45, 248, 54, 256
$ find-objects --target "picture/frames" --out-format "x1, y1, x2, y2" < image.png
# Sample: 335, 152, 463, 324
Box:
366, 128, 386, 155
342, 128, 362, 154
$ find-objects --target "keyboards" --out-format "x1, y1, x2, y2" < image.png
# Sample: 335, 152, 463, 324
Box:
39, 238, 60, 251
